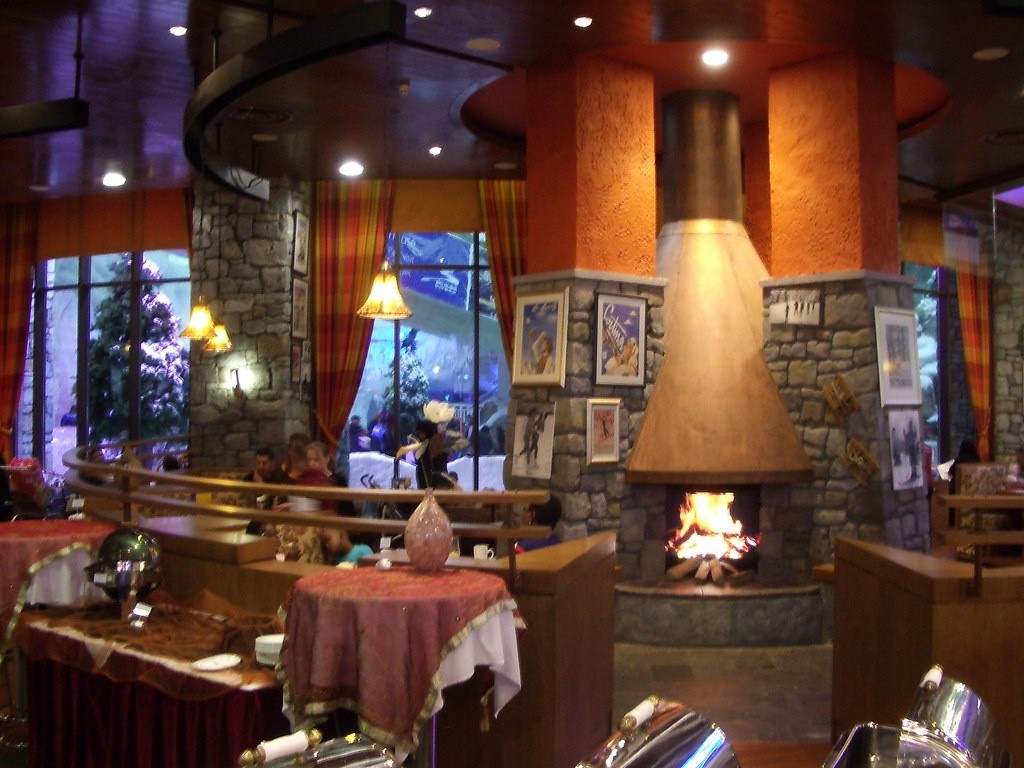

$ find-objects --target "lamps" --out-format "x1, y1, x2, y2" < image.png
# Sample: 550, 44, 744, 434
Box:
178, 173, 234, 354
356, 102, 411, 322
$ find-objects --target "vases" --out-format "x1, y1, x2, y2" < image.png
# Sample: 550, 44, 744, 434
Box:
404, 486, 454, 572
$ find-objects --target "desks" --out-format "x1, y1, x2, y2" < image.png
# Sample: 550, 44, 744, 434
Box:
278, 566, 527, 768
9, 585, 291, 768
1, 522, 115, 746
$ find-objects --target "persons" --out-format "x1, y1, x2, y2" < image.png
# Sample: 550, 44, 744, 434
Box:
514, 408, 547, 473
891, 420, 919, 482
416, 420, 463, 491
532, 332, 553, 375
1005, 448, 1024, 488
86, 448, 108, 486
517, 495, 561, 553
155, 456, 180, 486
349, 410, 404, 457
247, 433, 375, 567
924, 428, 981, 536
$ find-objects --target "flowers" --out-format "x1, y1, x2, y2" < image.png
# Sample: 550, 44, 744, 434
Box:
397, 433, 434, 490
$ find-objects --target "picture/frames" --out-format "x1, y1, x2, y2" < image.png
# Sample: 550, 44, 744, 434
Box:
585, 396, 621, 466
873, 303, 924, 408
594, 290, 649, 389
291, 278, 309, 339
293, 209, 311, 276
512, 284, 567, 389
